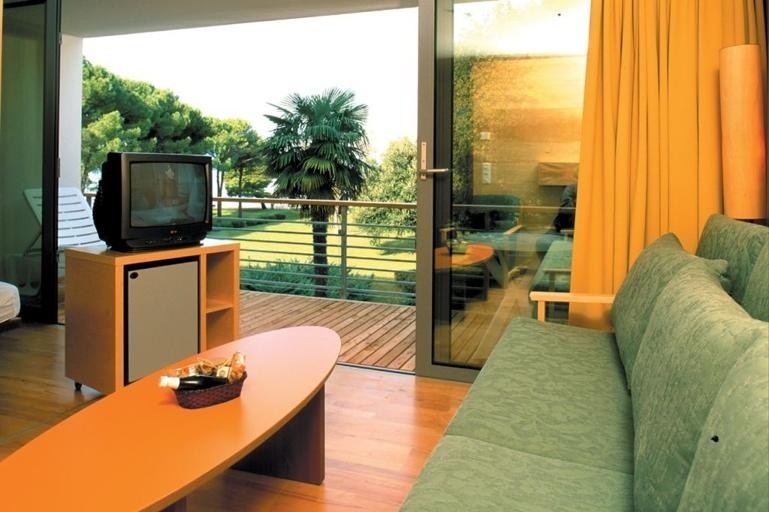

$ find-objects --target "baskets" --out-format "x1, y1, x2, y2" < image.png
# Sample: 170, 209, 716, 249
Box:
170, 359, 247, 409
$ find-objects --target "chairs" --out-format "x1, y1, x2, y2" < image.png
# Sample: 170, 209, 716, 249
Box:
461, 192, 526, 289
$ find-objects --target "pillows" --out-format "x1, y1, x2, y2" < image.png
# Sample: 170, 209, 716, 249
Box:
676, 336, 768, 511
632, 262, 765, 508
611, 230, 730, 391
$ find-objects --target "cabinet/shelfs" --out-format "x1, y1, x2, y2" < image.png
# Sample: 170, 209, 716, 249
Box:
62, 240, 242, 402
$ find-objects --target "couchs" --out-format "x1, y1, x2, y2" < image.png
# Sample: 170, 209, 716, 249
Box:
526, 225, 579, 321
390, 213, 769, 511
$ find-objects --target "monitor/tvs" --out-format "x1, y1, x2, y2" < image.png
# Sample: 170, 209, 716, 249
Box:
92, 151, 213, 251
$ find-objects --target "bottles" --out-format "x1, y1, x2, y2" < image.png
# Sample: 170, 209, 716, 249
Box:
157, 375, 228, 390
